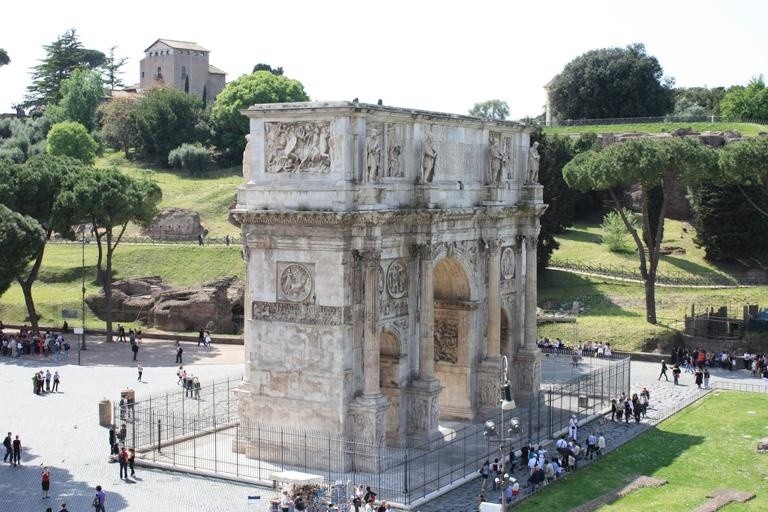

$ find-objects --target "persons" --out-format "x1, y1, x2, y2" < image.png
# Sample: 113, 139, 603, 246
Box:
611, 388, 649, 424
389, 145, 401, 177
281, 483, 393, 512
658, 346, 768, 389
503, 251, 513, 275
226, 234, 229, 246
198, 234, 202, 246
529, 141, 541, 185
41, 467, 51, 500
109, 394, 135, 479
176, 328, 210, 399
32, 369, 60, 395
366, 129, 380, 181
283, 269, 292, 290
422, 133, 436, 184
538, 337, 611, 368
388, 262, 403, 293
264, 122, 330, 172
61, 502, 66, 511
1, 321, 71, 360
115, 324, 143, 382
91, 486, 105, 511
3, 432, 21, 467
479, 415, 606, 504
489, 136, 502, 185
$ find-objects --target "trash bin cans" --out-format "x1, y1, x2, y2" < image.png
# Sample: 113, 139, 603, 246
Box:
736, 356, 745, 369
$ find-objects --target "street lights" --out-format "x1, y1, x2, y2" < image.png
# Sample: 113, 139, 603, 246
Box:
483, 353, 525, 511
80, 231, 94, 350
